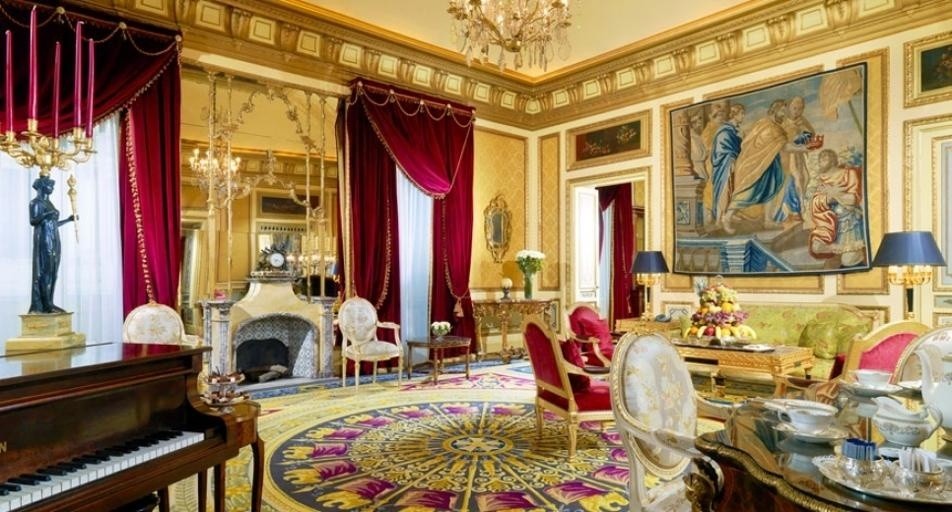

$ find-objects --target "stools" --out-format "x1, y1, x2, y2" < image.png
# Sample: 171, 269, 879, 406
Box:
406, 334, 470, 383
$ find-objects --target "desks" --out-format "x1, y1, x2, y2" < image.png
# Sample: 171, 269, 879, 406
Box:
157, 396, 267, 511
473, 297, 561, 362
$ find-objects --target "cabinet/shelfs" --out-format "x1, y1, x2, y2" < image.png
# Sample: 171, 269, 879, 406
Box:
617, 315, 673, 337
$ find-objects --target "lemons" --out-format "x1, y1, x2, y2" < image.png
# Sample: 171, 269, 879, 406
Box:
720, 328, 730, 337
720, 301, 732, 315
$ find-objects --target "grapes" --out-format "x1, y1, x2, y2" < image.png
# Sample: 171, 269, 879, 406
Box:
703, 311, 750, 324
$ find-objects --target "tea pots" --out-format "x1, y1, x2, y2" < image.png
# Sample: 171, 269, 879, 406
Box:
914, 346, 951, 442
872, 396, 941, 446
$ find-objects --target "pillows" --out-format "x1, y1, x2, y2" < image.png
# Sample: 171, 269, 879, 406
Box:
559, 337, 584, 370
828, 351, 847, 379
576, 317, 615, 360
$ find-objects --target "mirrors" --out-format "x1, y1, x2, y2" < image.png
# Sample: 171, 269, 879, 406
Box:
483, 195, 514, 264
258, 229, 337, 274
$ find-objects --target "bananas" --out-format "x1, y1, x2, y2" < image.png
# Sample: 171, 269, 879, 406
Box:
708, 302, 722, 315
697, 325, 707, 339
732, 301, 742, 311
715, 325, 721, 340
740, 323, 757, 341
683, 326, 690, 339
731, 325, 740, 339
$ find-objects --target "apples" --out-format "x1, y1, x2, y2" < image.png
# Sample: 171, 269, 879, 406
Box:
742, 330, 749, 338
704, 326, 715, 336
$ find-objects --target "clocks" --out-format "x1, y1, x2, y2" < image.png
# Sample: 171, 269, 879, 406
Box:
266, 243, 289, 273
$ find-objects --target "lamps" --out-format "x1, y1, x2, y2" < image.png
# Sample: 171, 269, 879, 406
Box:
454, 2, 571, 72
630, 249, 670, 322
287, 252, 335, 277
186, 73, 244, 186
499, 278, 514, 303
873, 229, 946, 321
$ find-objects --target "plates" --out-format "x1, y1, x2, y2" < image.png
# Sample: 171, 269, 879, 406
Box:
771, 421, 850, 445
899, 380, 924, 395
775, 438, 836, 458
838, 393, 902, 416
815, 454, 951, 503
846, 384, 903, 399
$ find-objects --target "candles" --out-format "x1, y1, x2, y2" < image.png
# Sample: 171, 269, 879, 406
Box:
5, 1, 100, 145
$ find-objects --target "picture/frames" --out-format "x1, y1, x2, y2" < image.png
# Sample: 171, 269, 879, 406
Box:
901, 30, 952, 108
567, 108, 653, 170
260, 194, 309, 216
659, 43, 893, 297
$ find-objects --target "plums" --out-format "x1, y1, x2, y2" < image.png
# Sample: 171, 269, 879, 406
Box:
691, 313, 701, 321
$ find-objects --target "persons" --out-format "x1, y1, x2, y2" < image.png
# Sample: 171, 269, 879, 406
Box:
29, 177, 79, 313
685, 94, 864, 270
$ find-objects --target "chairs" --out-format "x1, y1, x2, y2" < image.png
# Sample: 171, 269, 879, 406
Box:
566, 302, 616, 374
887, 327, 952, 383
337, 297, 403, 385
520, 315, 614, 458
123, 301, 197, 346
608, 327, 721, 511
844, 322, 929, 375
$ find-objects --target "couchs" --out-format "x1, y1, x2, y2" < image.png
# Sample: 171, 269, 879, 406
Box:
673, 302, 872, 395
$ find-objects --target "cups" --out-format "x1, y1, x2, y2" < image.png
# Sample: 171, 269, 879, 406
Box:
777, 453, 815, 474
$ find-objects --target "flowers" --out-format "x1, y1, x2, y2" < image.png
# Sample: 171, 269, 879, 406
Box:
431, 320, 450, 332
513, 248, 546, 288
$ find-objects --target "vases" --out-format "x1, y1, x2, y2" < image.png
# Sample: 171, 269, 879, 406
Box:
520, 274, 533, 298
434, 332, 447, 344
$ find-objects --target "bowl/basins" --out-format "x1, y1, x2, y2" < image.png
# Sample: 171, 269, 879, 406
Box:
777, 406, 836, 434
853, 366, 891, 384
